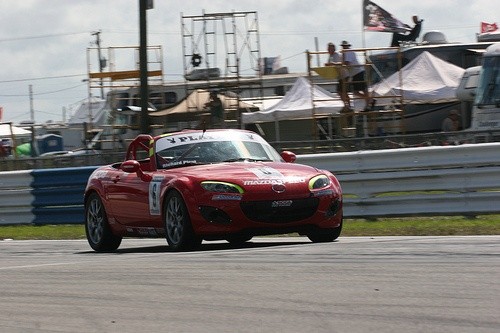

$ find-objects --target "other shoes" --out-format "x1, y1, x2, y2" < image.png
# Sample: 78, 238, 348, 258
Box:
341, 106, 353, 113
361, 107, 372, 112
370, 99, 376, 108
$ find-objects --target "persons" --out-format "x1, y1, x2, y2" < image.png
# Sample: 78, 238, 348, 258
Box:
325, 40, 376, 113
205, 92, 224, 122
391, 16, 422, 48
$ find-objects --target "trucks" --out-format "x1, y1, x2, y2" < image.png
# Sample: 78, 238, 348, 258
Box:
1, 29, 500, 157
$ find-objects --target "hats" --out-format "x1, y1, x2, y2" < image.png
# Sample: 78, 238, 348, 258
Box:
340, 40, 351, 45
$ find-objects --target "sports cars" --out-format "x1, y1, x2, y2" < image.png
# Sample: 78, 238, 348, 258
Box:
83, 129, 344, 253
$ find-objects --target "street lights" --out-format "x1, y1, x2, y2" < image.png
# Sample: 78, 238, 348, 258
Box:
82, 78, 105, 99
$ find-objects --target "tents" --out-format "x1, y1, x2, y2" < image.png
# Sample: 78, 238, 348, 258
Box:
241, 76, 354, 129
354, 50, 466, 110
148, 88, 259, 121
